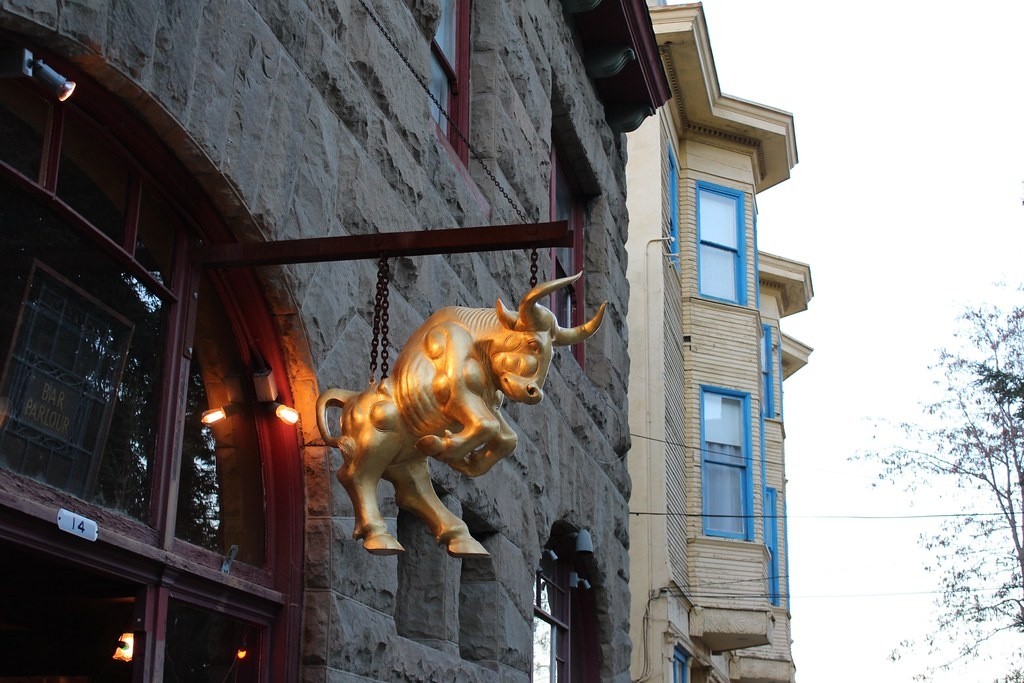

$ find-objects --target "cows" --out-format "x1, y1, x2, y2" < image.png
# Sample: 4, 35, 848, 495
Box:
315, 270, 609, 558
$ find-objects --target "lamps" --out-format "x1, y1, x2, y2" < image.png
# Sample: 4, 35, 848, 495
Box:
112, 633, 135, 662
569, 571, 591, 590
536, 549, 559, 574
566, 529, 593, 554
28, 58, 76, 102
